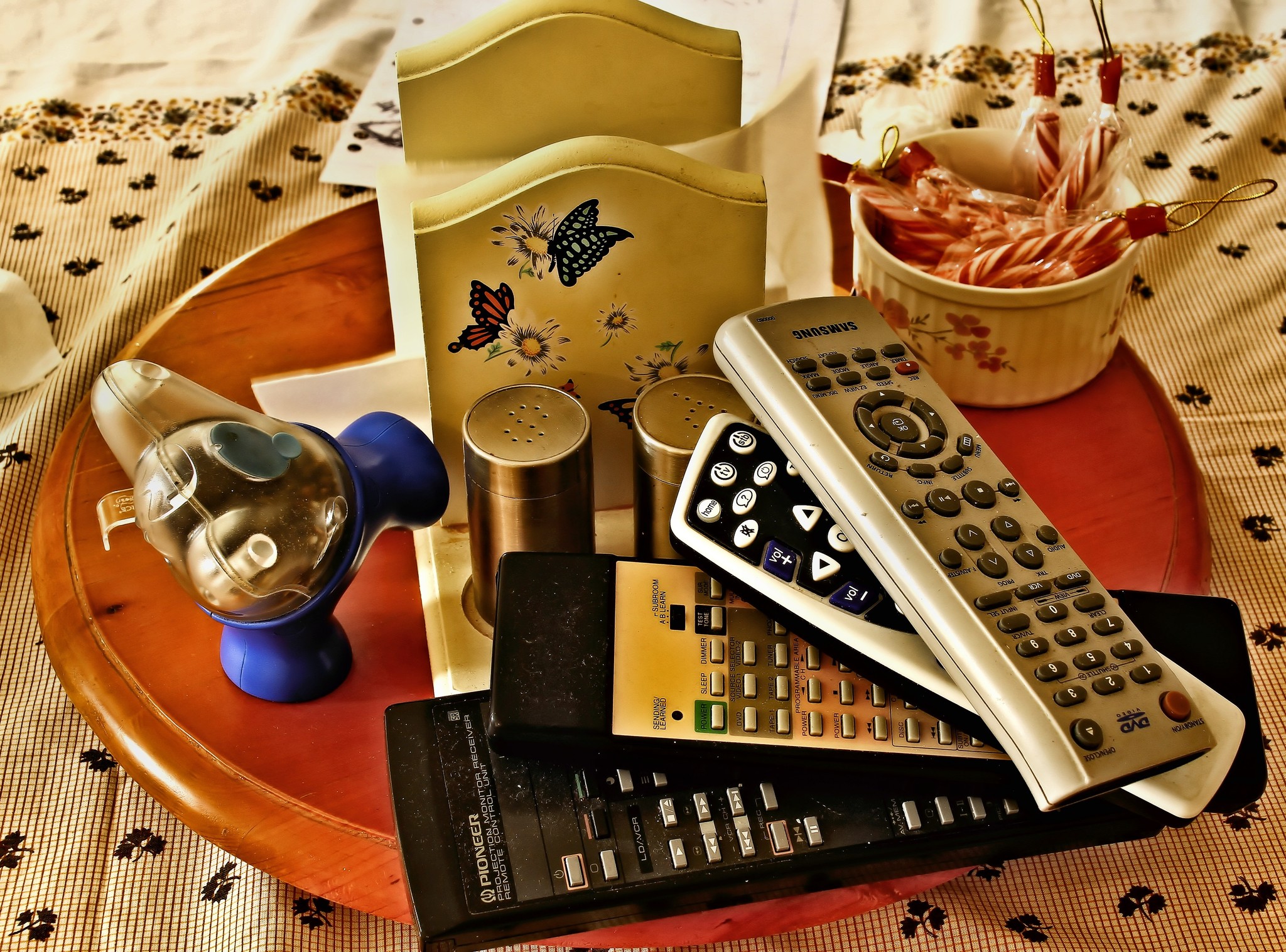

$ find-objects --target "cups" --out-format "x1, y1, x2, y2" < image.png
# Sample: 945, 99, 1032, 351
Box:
851, 127, 1143, 407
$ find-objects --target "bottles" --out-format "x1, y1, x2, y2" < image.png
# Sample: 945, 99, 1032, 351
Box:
633, 374, 766, 560
462, 380, 597, 639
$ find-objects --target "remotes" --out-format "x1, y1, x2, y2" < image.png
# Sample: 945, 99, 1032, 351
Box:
384, 297, 1267, 952
